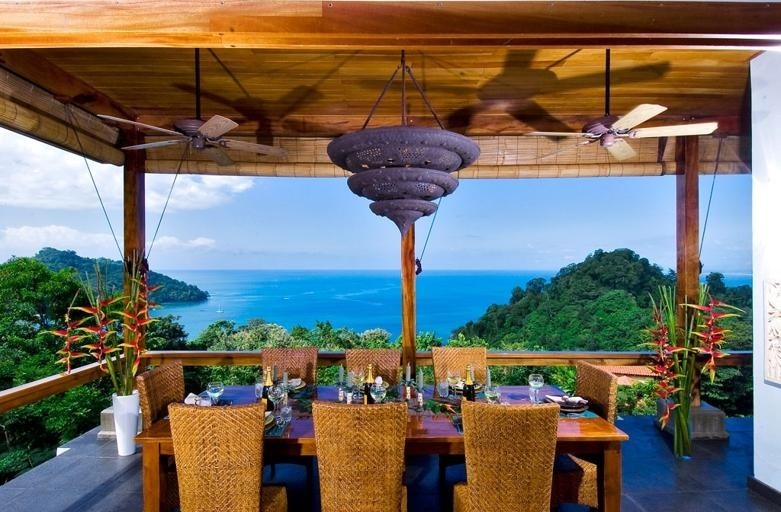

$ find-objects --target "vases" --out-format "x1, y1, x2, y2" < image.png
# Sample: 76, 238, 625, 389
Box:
637, 283, 745, 458
48, 249, 163, 395
112, 389, 140, 456
673, 406, 696, 463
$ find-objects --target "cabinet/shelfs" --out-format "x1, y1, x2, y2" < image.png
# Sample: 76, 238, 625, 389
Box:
263, 366, 277, 412
363, 362, 377, 403
337, 366, 346, 402
347, 371, 355, 403
280, 372, 290, 407
415, 391, 426, 429
465, 365, 475, 401
402, 364, 415, 423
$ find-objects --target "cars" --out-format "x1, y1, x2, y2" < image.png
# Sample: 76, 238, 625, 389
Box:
254, 383, 263, 399
280, 406, 292, 422
438, 380, 449, 397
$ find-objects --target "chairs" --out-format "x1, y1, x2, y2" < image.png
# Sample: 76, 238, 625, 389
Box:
552, 360, 617, 511
261, 347, 488, 482
137, 360, 186, 510
312, 399, 407, 511
454, 401, 560, 511
168, 401, 288, 511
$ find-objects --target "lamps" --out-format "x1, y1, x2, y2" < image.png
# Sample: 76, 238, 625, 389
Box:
326, 50, 481, 240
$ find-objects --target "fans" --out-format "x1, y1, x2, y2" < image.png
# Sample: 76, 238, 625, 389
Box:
523, 48, 719, 163
96, 48, 287, 166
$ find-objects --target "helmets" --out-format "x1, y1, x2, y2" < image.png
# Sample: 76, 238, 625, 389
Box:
263, 419, 277, 432
449, 382, 483, 391
558, 408, 590, 413
449, 385, 486, 394
263, 415, 275, 426
275, 379, 307, 391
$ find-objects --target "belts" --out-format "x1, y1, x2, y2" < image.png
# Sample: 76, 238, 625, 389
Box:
484, 383, 500, 404
447, 368, 461, 397
370, 383, 387, 403
268, 385, 284, 414
206, 381, 225, 406
529, 373, 545, 403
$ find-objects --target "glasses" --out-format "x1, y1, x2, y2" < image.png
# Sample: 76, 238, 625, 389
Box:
326, 50, 481, 240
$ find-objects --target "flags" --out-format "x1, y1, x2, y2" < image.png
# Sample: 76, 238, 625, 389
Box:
673, 406, 696, 463
112, 389, 140, 456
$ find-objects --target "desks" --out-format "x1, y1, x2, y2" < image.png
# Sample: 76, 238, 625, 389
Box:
137, 384, 629, 511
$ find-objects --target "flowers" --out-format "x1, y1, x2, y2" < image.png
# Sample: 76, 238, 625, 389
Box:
48, 249, 163, 395
637, 283, 745, 458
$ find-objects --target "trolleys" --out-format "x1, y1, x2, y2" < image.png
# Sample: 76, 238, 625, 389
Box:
523, 48, 719, 163
96, 48, 287, 166
